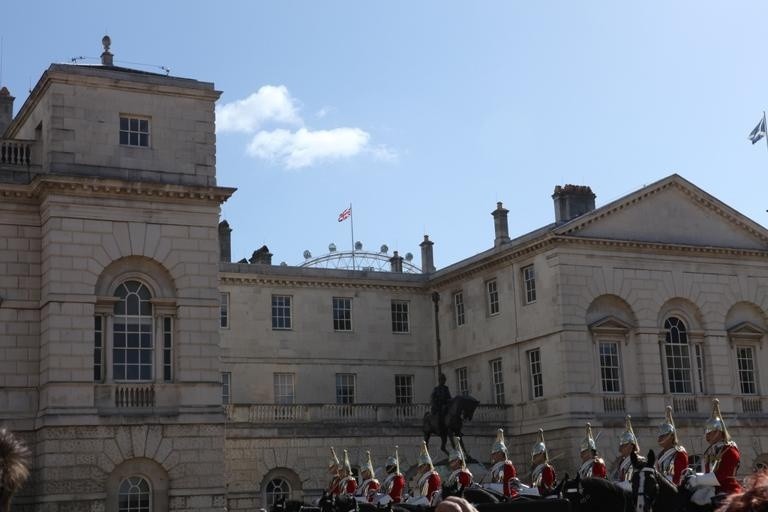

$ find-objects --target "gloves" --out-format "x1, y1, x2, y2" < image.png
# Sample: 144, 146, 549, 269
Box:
476, 482, 504, 494
510, 477, 530, 488
350, 493, 431, 506
615, 480, 632, 492
680, 472, 721, 489
517, 487, 540, 496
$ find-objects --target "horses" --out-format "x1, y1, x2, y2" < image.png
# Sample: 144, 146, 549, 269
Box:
422, 395, 480, 463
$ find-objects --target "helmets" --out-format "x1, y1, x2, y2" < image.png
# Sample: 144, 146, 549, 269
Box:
656, 424, 676, 438
329, 456, 351, 471
532, 442, 546, 456
491, 443, 507, 454
360, 463, 373, 472
448, 450, 463, 463
417, 456, 432, 467
705, 417, 725, 434
618, 431, 635, 446
384, 456, 398, 467
581, 438, 596, 452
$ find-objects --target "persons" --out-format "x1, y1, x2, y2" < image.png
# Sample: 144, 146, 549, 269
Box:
0, 429, 32, 511
429, 373, 453, 435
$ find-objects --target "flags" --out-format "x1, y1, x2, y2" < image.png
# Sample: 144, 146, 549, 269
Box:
747, 115, 766, 145
337, 206, 352, 222
